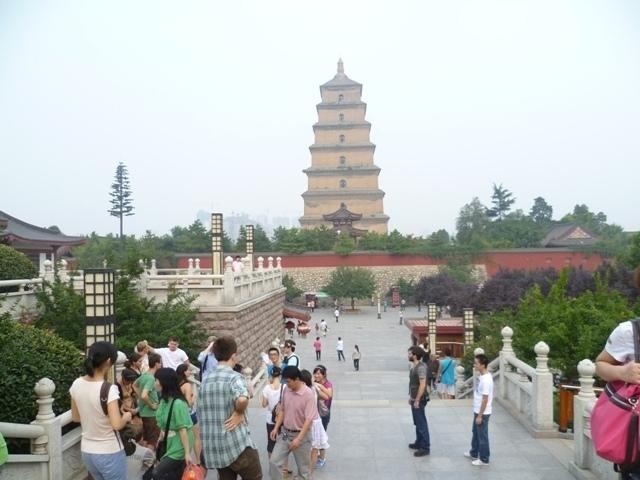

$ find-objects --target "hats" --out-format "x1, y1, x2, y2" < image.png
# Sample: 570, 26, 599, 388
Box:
279, 342, 296, 348
271, 367, 281, 376
122, 368, 139, 377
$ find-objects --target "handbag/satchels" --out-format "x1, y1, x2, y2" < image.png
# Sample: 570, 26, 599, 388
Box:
272, 383, 283, 423
319, 399, 329, 417
155, 395, 177, 461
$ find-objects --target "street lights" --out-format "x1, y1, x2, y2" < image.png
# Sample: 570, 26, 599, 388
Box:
83, 268, 116, 385
246, 225, 254, 271
462, 308, 474, 356
427, 303, 436, 354
211, 213, 224, 285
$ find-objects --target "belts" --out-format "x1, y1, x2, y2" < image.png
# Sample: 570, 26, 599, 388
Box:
282, 425, 301, 432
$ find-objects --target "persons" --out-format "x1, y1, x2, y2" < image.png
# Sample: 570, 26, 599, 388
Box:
260, 367, 293, 476
426, 354, 439, 394
407, 346, 430, 458
300, 369, 330, 474
268, 365, 318, 479
195, 336, 264, 480
267, 346, 287, 384
313, 365, 333, 469
68, 341, 132, 479
121, 352, 143, 387
351, 344, 361, 372
281, 339, 301, 370
148, 336, 190, 372
143, 368, 196, 479
335, 337, 346, 363
176, 363, 203, 467
319, 319, 328, 338
313, 336, 323, 361
310, 298, 316, 313
119, 423, 156, 480
136, 341, 149, 374
132, 354, 162, 450
116, 368, 143, 443
334, 308, 339, 323
433, 348, 456, 399
463, 354, 494, 466
198, 336, 218, 382
232, 254, 245, 274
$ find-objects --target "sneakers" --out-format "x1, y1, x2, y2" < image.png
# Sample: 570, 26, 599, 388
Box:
283, 469, 292, 477
316, 456, 326, 469
471, 458, 489, 466
414, 448, 430, 457
464, 451, 477, 460
409, 444, 418, 449
